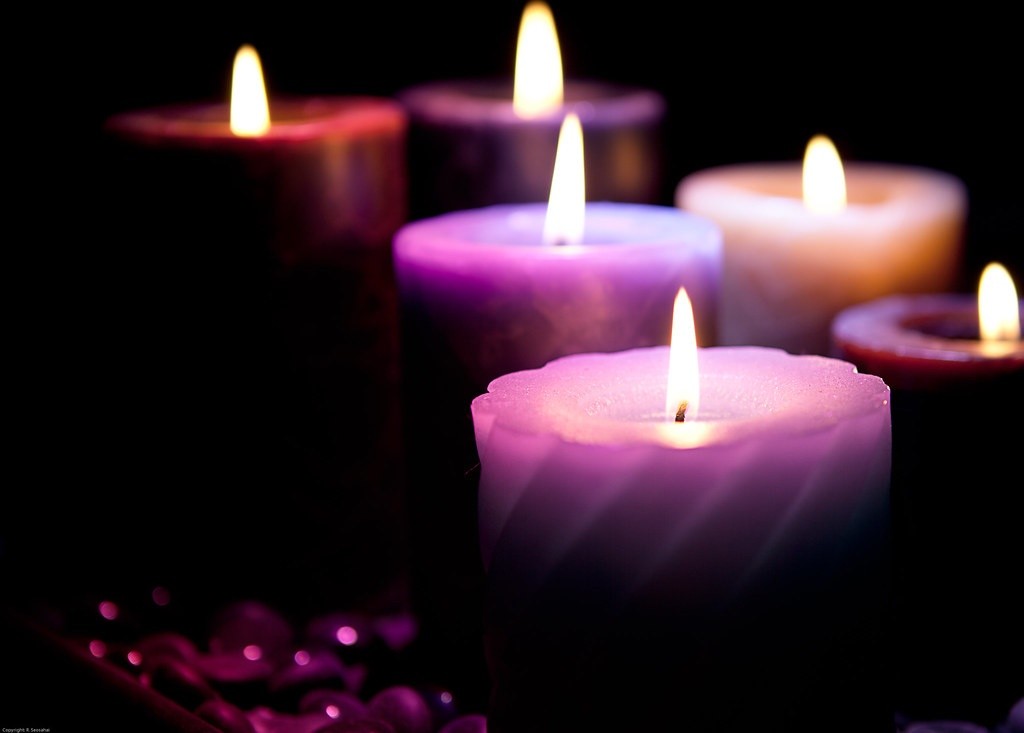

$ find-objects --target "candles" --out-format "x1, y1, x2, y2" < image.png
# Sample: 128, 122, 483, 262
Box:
105, 0, 1024, 581
